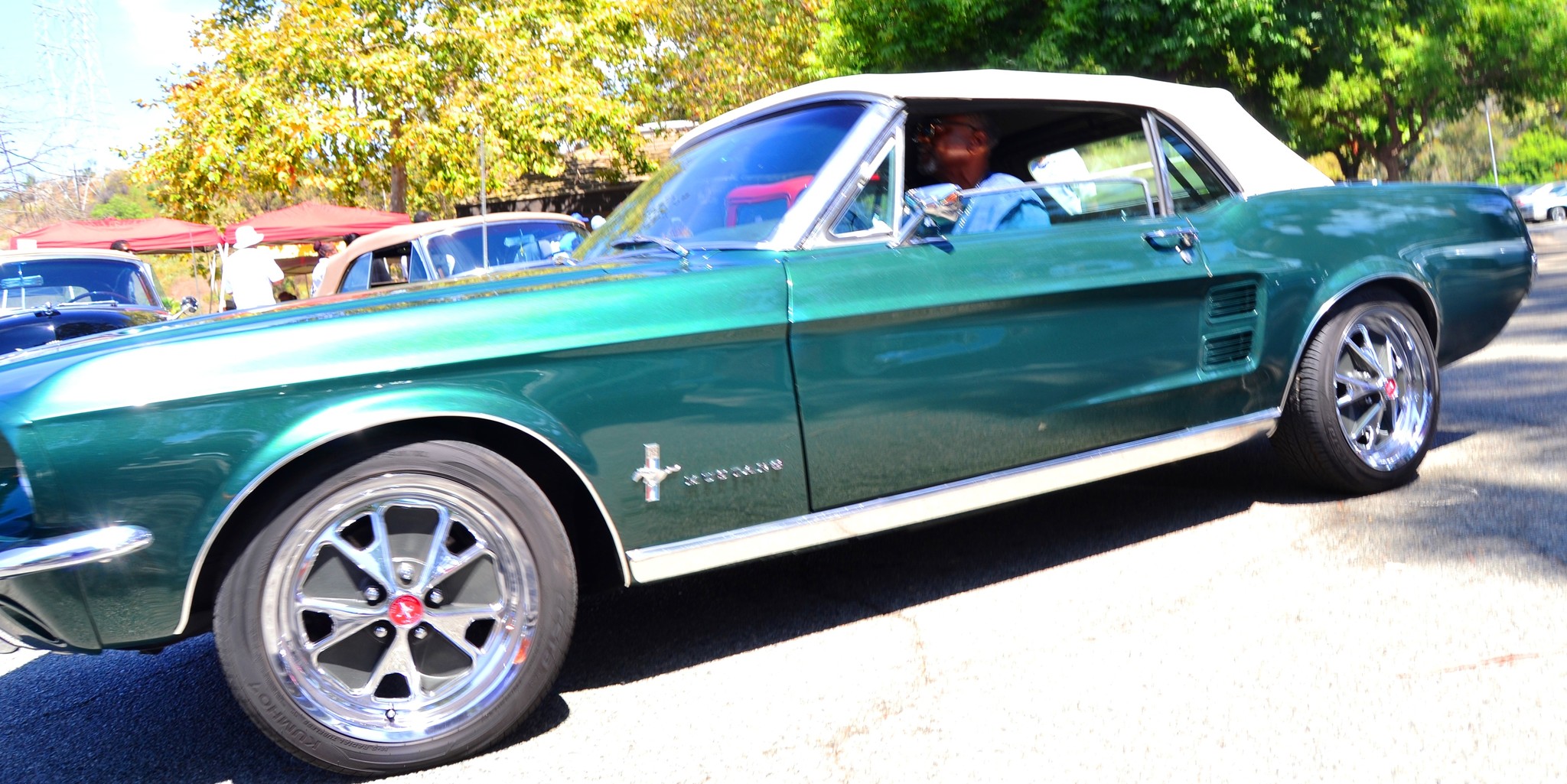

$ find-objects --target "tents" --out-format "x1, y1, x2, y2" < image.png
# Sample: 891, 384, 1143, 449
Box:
10, 216, 225, 318
222, 199, 415, 301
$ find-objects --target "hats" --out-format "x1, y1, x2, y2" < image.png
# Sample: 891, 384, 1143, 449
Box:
232, 225, 264, 250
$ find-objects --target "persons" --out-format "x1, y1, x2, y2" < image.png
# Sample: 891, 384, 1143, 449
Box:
835, 109, 1053, 237
109, 240, 137, 259
310, 240, 338, 299
223, 225, 284, 311
343, 233, 393, 283
411, 209, 474, 282
511, 212, 691, 264
278, 291, 293, 302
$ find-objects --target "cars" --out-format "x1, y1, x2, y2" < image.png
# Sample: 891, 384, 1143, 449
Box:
1, 250, 199, 359
1511, 180, 1567, 225
312, 210, 591, 299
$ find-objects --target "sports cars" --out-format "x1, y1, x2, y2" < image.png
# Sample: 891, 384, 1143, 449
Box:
0, 59, 1540, 777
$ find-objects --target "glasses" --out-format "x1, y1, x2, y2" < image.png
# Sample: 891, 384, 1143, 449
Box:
123, 249, 136, 253
